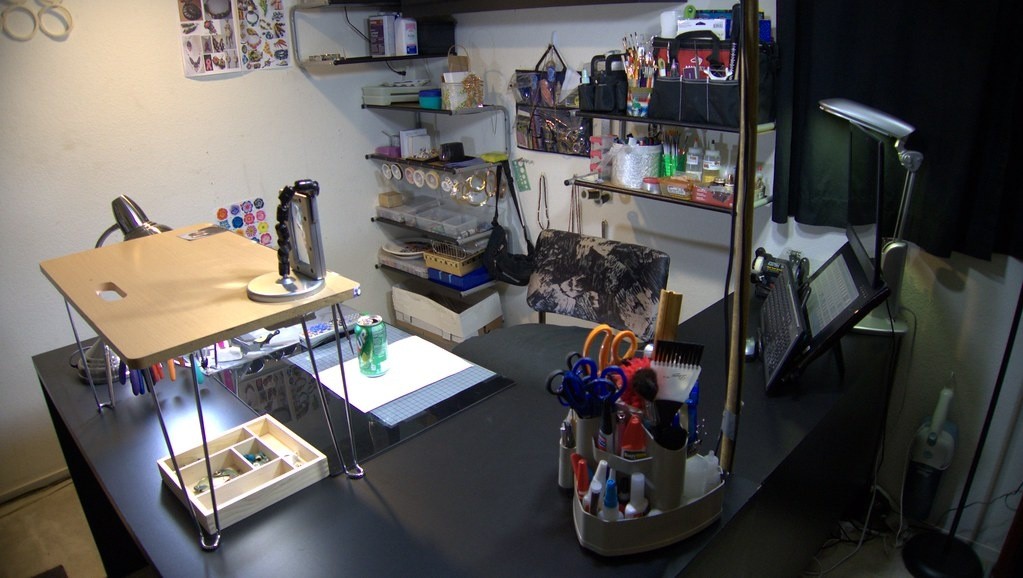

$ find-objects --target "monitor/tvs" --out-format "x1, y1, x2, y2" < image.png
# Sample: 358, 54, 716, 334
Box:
798, 239, 891, 370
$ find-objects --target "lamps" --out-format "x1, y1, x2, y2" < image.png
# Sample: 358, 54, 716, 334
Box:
819, 96, 925, 335
68, 193, 174, 385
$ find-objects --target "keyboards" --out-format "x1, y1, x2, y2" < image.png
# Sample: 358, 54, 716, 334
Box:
761, 262, 804, 391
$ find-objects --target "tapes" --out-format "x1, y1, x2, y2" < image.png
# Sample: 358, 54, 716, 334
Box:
425, 171, 440, 190
404, 167, 416, 184
391, 164, 403, 181
412, 169, 424, 187
381, 163, 393, 180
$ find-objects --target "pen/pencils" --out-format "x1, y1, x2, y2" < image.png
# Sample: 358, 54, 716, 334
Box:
558, 410, 578, 448
613, 122, 690, 155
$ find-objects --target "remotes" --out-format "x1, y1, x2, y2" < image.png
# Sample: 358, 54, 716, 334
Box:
298, 311, 370, 348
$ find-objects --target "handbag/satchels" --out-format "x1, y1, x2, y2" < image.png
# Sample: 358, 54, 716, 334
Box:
483, 160, 534, 286
516, 42, 581, 110
652, 30, 732, 80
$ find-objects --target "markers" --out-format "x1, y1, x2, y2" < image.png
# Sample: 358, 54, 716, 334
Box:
570, 452, 602, 515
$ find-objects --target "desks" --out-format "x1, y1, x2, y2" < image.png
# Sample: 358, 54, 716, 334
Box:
37, 220, 367, 551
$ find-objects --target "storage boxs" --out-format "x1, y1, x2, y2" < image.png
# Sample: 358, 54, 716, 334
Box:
392, 276, 504, 352
374, 190, 478, 240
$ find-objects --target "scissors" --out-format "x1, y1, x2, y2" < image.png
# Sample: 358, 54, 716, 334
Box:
544, 322, 638, 419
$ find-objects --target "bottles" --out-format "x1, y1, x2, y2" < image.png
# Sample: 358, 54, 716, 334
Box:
702, 140, 721, 185
687, 139, 703, 180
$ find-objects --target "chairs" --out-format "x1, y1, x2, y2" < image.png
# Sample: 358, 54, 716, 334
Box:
452, 229, 671, 384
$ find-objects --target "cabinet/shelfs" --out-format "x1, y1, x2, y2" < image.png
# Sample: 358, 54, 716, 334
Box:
361, 104, 512, 297
288, 1, 455, 67
564, 108, 775, 212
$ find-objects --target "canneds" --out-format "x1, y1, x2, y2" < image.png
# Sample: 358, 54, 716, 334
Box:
354, 314, 388, 378
